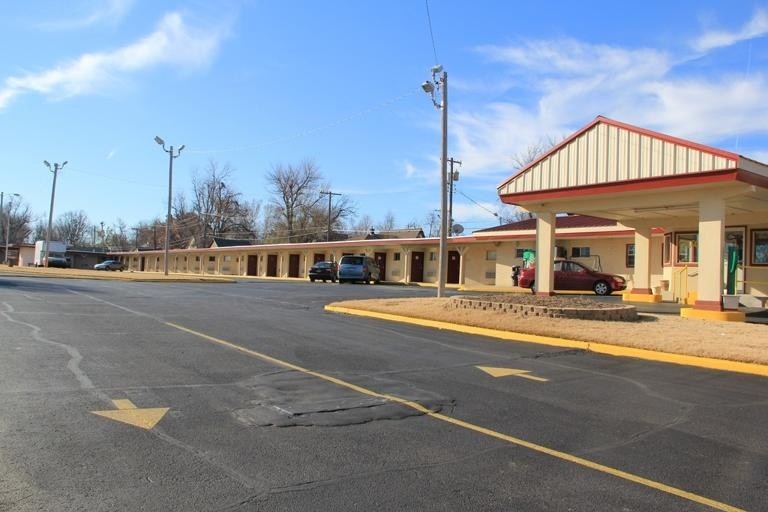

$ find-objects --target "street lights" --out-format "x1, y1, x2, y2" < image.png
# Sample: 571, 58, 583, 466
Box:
0, 191, 20, 264
420, 63, 451, 299
154, 136, 185, 274
43, 160, 68, 268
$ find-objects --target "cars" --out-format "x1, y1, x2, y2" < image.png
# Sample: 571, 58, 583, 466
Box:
310, 261, 338, 284
516, 260, 629, 296
94, 261, 128, 272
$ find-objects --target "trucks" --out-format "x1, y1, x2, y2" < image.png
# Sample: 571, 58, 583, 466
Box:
34, 240, 67, 268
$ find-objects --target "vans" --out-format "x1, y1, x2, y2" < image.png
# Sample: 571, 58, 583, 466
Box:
336, 254, 382, 284
